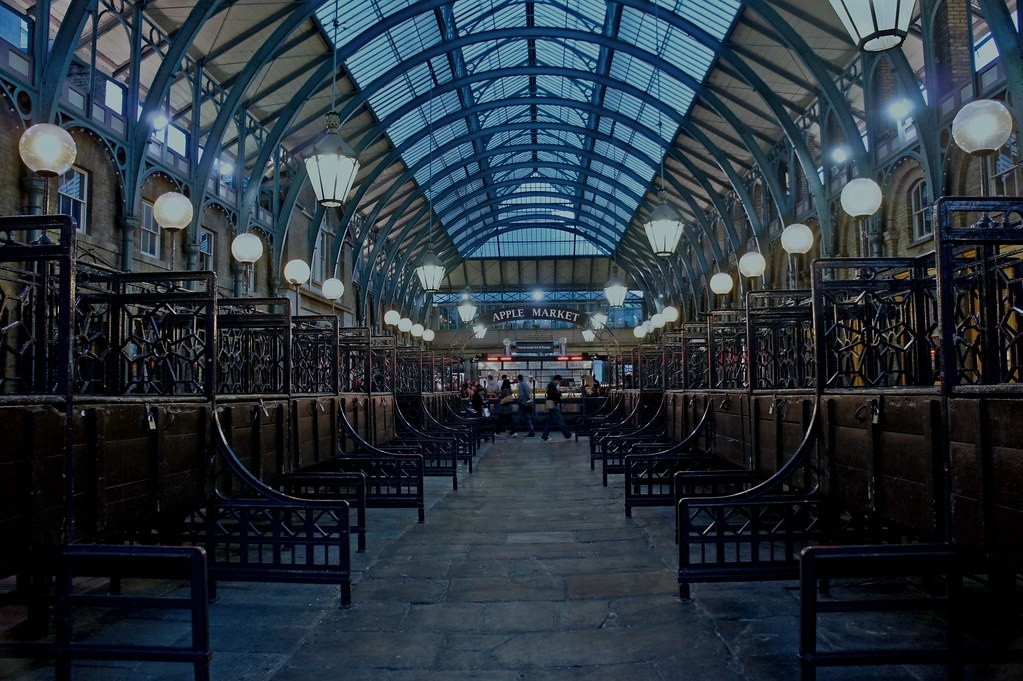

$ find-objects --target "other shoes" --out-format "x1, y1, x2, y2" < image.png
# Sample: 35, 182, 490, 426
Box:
524, 434, 535, 437
509, 430, 514, 434
494, 433, 500, 438
510, 432, 518, 437
541, 437, 552, 441
570, 433, 575, 439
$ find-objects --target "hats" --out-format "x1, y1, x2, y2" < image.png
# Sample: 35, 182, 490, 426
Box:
554, 375, 563, 380
528, 375, 534, 378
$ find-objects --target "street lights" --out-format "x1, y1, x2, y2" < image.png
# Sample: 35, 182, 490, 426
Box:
16, 120, 78, 384
150, 191, 198, 388
952, 98, 1013, 372
283, 260, 310, 395
384, 311, 435, 396
322, 279, 344, 391
231, 229, 265, 386
781, 223, 816, 386
631, 306, 680, 382
841, 178, 882, 371
708, 274, 734, 398
740, 250, 765, 383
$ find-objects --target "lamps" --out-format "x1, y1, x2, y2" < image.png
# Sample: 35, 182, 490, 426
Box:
20, 0, 1023, 343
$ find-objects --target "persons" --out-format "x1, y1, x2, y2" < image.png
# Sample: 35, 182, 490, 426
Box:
585, 375, 599, 396
494, 386, 519, 438
526, 375, 539, 389
501, 375, 511, 390
486, 375, 500, 392
510, 375, 535, 438
541, 375, 575, 441
445, 379, 485, 415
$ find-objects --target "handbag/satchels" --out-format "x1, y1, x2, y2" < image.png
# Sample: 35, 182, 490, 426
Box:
523, 399, 534, 412
544, 400, 555, 409
